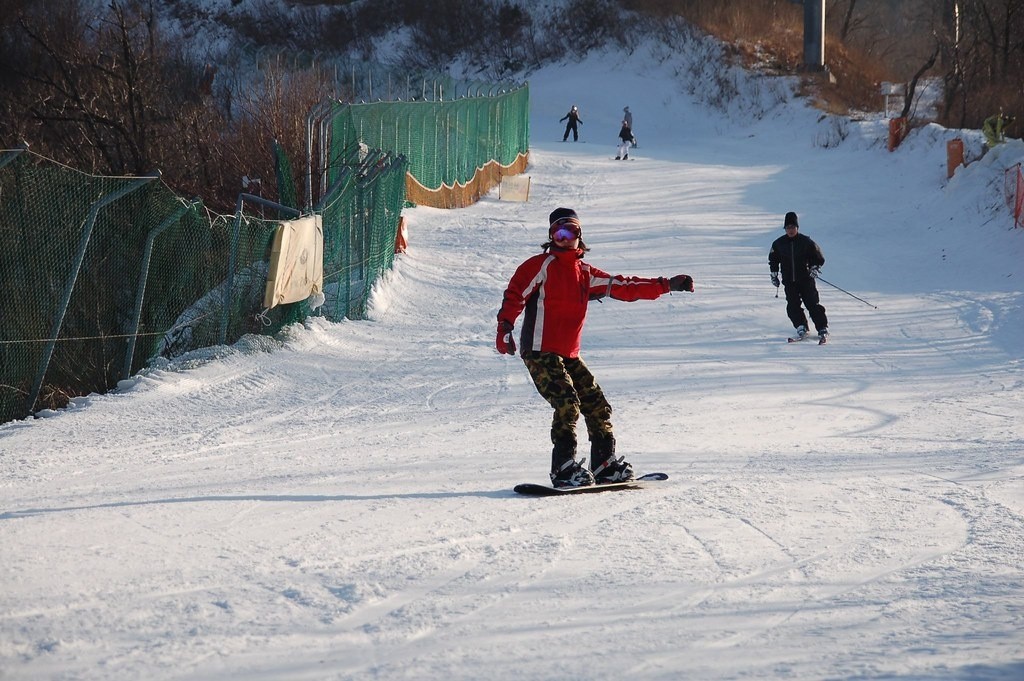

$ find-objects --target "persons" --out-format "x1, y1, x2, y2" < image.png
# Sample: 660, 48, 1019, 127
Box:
768, 212, 830, 346
559, 105, 583, 142
615, 105, 637, 160
496, 207, 695, 488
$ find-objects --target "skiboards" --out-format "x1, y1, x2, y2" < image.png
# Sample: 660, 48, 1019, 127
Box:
785, 329, 830, 347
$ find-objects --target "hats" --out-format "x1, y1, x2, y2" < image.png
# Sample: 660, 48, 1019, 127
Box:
624, 107, 629, 112
549, 208, 580, 227
571, 105, 578, 111
783, 212, 798, 226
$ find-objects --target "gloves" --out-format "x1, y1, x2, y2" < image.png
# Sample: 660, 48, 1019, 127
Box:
771, 272, 780, 288
670, 275, 694, 292
495, 323, 517, 355
810, 265, 823, 278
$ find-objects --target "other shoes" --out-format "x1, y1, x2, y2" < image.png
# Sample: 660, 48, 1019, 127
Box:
797, 326, 807, 336
615, 157, 620, 160
818, 328, 829, 338
623, 154, 628, 160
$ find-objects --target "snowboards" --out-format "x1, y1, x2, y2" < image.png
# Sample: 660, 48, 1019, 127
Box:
555, 139, 588, 144
510, 471, 668, 496
608, 157, 636, 162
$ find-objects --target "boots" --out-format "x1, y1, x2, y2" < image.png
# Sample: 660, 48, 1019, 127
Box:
551, 448, 594, 485
591, 439, 633, 482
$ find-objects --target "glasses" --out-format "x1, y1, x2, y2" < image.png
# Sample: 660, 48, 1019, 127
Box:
549, 223, 580, 242
784, 224, 798, 229
